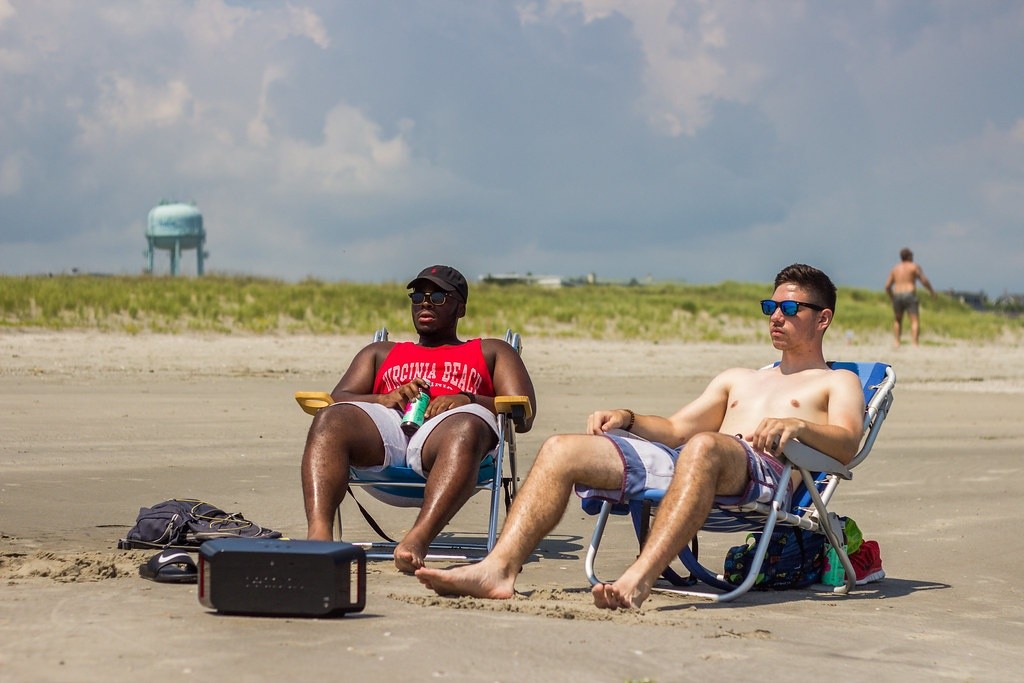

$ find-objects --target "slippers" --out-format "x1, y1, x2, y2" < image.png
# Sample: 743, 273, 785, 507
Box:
138, 548, 197, 582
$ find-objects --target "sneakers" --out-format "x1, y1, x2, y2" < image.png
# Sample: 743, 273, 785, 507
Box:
844, 539, 887, 586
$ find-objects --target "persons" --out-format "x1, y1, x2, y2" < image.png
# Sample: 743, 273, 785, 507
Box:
411, 262, 868, 611
885, 248, 938, 349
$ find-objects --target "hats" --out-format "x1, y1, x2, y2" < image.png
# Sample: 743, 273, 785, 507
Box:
405, 265, 468, 304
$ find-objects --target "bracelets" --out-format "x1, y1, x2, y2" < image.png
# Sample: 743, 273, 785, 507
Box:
623, 408, 635, 431
458, 391, 476, 404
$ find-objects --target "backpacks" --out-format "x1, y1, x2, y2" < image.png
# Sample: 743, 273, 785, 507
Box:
117, 497, 284, 556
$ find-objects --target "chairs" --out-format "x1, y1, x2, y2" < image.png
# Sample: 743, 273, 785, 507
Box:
583, 361, 897, 603
332, 328, 522, 563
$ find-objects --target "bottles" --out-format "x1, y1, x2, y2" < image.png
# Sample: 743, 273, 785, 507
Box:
820, 514, 848, 586
398, 377, 432, 437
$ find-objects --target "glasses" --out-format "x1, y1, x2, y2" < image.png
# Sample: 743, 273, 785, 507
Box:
407, 292, 463, 306
760, 300, 824, 317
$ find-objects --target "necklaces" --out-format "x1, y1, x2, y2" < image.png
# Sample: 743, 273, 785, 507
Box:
300, 265, 538, 579
